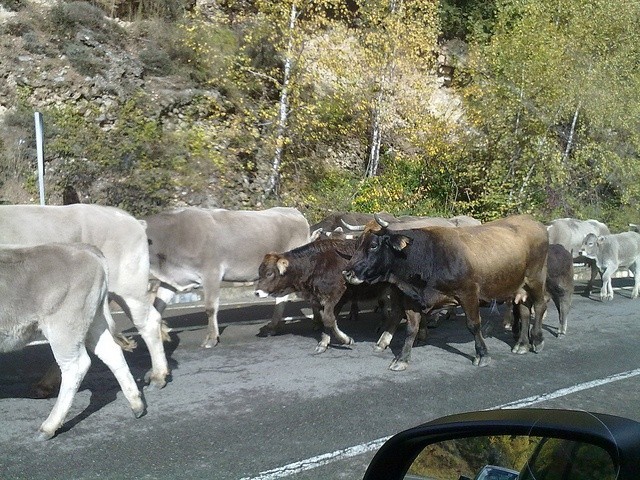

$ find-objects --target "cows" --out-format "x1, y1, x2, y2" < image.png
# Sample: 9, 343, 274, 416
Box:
544, 243, 576, 338
0, 203, 173, 391
310, 211, 395, 234
340, 212, 549, 371
134, 206, 322, 349
0, 241, 145, 442
578, 223, 640, 301
254, 239, 430, 354
501, 297, 519, 331
544, 217, 611, 296
309, 215, 482, 329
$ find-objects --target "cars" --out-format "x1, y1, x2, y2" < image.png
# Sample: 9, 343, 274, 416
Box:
518, 434, 616, 480
459, 465, 519, 479
